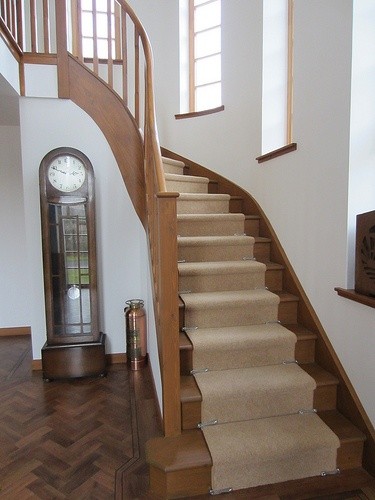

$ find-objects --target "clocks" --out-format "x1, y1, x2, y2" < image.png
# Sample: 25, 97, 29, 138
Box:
45, 152, 87, 194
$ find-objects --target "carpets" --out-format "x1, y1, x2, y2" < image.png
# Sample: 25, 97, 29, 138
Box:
162, 155, 341, 497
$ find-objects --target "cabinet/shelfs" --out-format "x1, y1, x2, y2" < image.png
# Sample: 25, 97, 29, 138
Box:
38, 146, 108, 383
